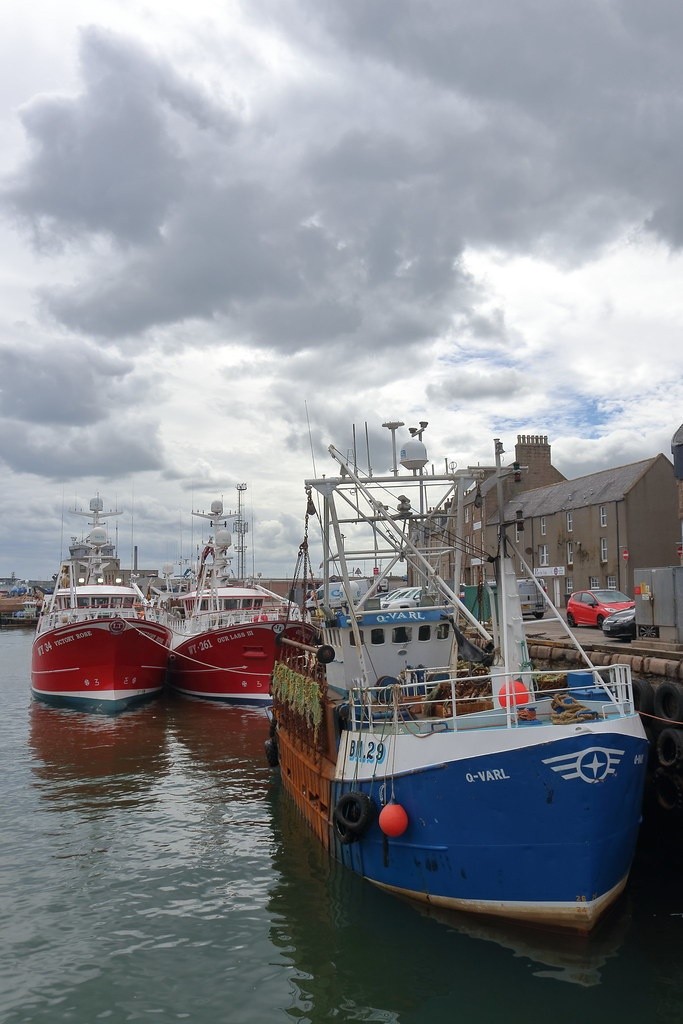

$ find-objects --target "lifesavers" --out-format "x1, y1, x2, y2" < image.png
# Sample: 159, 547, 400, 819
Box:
337, 791, 378, 830
264, 738, 279, 768
332, 807, 361, 845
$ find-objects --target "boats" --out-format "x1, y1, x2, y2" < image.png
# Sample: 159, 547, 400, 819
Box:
264, 396, 653, 938
29, 479, 174, 714
153, 479, 313, 707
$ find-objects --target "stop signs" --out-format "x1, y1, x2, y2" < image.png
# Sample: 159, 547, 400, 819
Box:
623, 550, 630, 561
676, 546, 683, 556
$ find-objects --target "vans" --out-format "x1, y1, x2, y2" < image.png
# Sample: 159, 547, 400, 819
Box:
486, 577, 548, 620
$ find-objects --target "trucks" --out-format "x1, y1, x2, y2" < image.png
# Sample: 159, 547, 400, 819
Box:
303, 579, 371, 618
377, 579, 389, 593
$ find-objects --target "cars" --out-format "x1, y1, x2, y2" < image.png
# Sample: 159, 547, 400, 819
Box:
566, 588, 636, 630
602, 605, 659, 643
402, 574, 408, 582
370, 580, 467, 610
